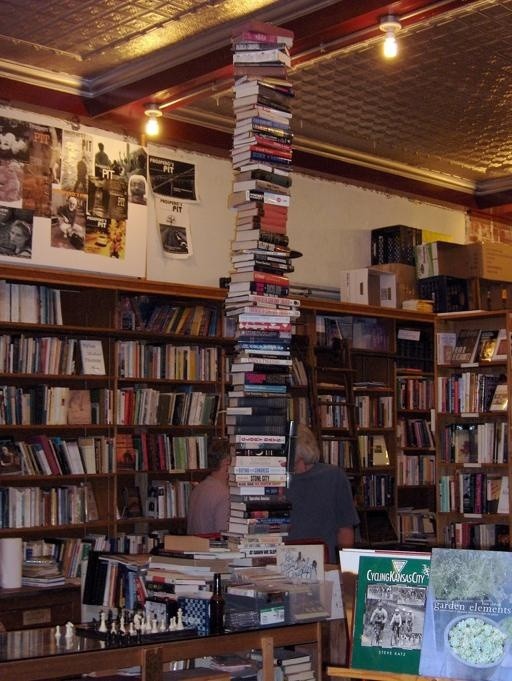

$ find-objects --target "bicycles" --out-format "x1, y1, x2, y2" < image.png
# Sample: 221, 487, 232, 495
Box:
369, 620, 385, 646
389, 619, 414, 646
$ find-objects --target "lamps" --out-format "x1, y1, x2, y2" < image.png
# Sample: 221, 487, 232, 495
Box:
377, 16, 403, 60
144, 103, 166, 138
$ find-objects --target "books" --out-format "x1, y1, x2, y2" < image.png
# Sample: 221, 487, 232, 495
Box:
0, 279, 512, 680
224, 21, 302, 288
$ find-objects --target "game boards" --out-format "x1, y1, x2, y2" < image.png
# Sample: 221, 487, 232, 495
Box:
75, 618, 198, 643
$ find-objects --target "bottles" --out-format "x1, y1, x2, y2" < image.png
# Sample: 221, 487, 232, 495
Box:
208, 573, 226, 634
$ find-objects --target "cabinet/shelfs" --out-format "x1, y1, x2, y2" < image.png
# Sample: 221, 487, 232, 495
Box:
0, 263, 448, 681
429, 309, 512, 552
2, 618, 333, 680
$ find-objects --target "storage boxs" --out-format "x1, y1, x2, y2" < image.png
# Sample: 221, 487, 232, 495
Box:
333, 221, 511, 310
221, 587, 291, 633
244, 575, 335, 624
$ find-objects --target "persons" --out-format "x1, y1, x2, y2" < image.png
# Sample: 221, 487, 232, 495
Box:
1, 116, 147, 260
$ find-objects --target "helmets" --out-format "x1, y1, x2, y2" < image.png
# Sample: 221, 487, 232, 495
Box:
377, 602, 383, 607
395, 607, 412, 613
